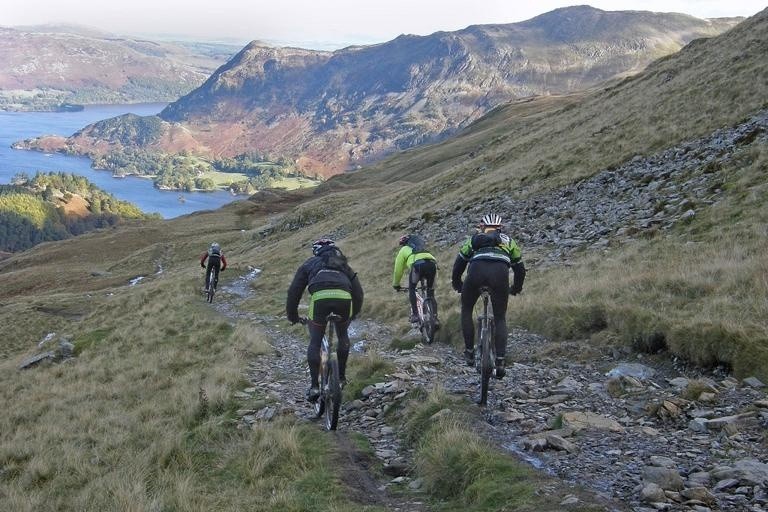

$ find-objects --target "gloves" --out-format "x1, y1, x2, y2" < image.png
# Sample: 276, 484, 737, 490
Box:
510, 284, 523, 295
451, 276, 463, 294
392, 285, 402, 293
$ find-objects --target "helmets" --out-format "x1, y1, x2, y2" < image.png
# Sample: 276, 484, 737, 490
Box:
311, 237, 335, 253
212, 242, 219, 246
478, 212, 503, 227
399, 234, 411, 244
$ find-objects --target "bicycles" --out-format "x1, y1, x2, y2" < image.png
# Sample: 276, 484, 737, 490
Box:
291, 314, 362, 429
200, 263, 225, 303
457, 283, 524, 408
392, 276, 438, 343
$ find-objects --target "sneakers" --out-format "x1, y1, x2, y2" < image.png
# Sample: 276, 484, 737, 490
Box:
407, 313, 420, 323
494, 359, 506, 379
304, 387, 321, 403
463, 349, 476, 367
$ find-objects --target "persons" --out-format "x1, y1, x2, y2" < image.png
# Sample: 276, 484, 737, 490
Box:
286, 239, 363, 403
392, 234, 440, 333
452, 213, 526, 380
201, 242, 226, 293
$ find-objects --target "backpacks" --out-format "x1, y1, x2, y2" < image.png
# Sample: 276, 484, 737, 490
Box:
315, 242, 347, 272
470, 228, 502, 251
211, 246, 221, 254
405, 235, 428, 254
